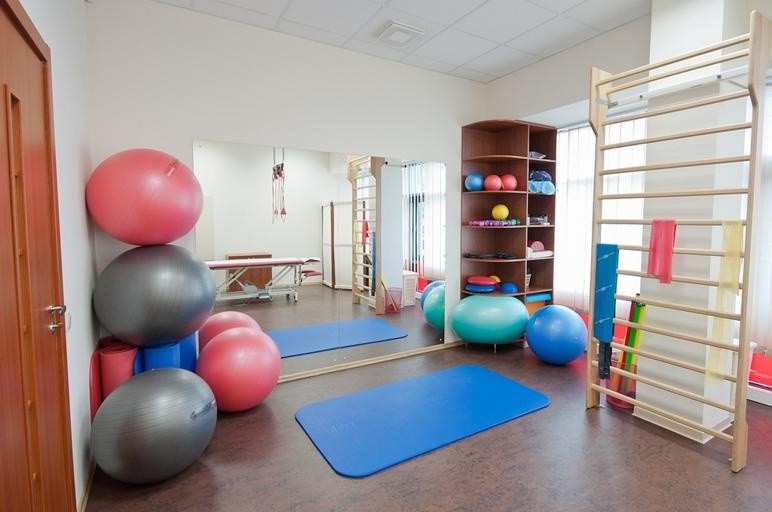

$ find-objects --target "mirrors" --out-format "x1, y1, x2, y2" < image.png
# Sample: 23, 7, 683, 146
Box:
192, 140, 446, 378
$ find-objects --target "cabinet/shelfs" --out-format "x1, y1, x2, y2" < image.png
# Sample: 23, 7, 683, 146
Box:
461, 119, 557, 354
403, 271, 419, 307
224, 253, 273, 292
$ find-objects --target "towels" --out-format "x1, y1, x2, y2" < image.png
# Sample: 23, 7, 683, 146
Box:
646, 218, 677, 284
592, 243, 619, 343
362, 219, 369, 245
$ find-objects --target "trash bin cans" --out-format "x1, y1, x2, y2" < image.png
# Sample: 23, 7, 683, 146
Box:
385, 287, 402, 313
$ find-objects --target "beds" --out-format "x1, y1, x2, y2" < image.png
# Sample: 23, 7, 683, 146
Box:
205, 257, 324, 307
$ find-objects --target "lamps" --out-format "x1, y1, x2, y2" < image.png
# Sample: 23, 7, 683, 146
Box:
377, 20, 424, 50
